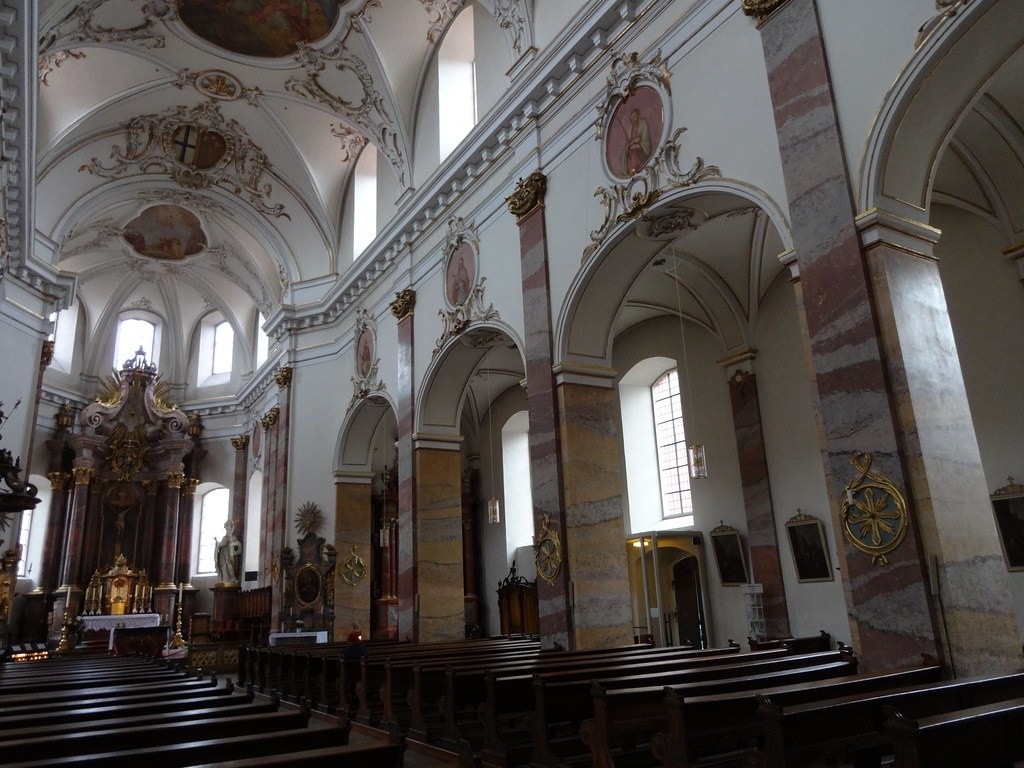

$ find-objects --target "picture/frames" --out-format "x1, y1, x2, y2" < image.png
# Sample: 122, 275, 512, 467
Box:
784, 508, 834, 583
989, 476, 1024, 572
709, 520, 750, 587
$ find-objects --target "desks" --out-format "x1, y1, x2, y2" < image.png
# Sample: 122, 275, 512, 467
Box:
82, 614, 160, 638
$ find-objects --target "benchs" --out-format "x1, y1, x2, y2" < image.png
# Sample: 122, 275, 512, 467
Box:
0, 651, 410, 768
233, 633, 1024, 768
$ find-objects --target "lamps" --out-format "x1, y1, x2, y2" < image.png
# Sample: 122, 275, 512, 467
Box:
486, 353, 500, 524
669, 243, 707, 480
380, 411, 389, 547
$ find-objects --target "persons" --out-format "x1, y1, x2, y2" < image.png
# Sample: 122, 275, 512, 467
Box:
212, 518, 243, 585
346, 620, 362, 643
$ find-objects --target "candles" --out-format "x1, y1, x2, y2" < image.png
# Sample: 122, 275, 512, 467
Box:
85, 588, 89, 602
142, 586, 146, 598
179, 583, 183, 603
99, 585, 103, 599
846, 489, 852, 504
66, 586, 71, 608
149, 586, 152, 599
135, 585, 138, 597
92, 588, 96, 600
